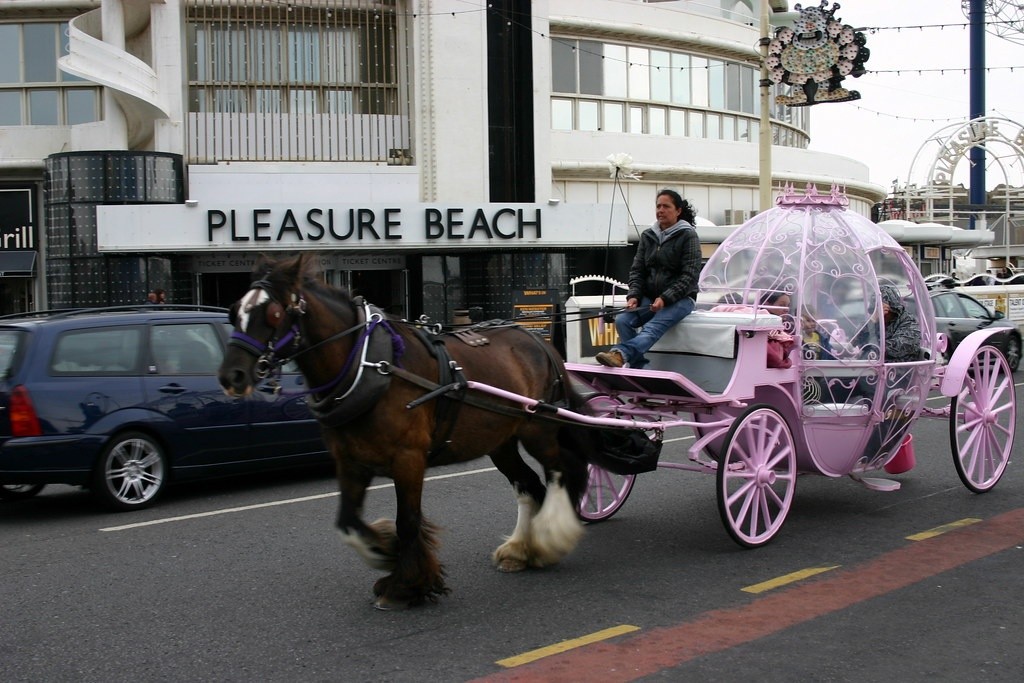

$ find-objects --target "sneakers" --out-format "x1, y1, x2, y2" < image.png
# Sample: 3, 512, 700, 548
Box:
596, 350, 624, 367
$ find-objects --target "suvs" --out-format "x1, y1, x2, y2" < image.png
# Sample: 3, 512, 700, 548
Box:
0, 304, 333, 513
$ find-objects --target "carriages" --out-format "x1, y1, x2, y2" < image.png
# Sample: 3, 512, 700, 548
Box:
216, 177, 1017, 613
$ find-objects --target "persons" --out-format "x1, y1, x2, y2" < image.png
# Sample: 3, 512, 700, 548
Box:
849, 285, 922, 458
141, 293, 157, 311
594, 189, 702, 369
759, 283, 848, 403
154, 288, 166, 304
939, 270, 1024, 290
793, 303, 836, 360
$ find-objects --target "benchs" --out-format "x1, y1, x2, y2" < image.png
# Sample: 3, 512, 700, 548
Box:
803, 404, 870, 474
562, 314, 782, 410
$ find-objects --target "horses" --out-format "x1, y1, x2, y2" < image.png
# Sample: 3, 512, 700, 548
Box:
218, 248, 593, 613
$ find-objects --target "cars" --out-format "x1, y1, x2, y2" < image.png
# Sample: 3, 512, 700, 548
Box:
836, 289, 1022, 372
